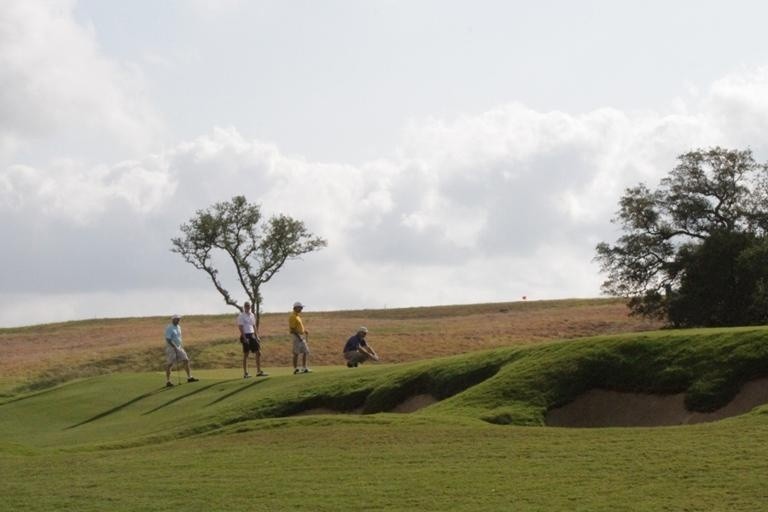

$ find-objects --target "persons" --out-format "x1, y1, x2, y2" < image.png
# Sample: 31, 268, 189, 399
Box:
342, 327, 379, 367
164, 313, 200, 391
236, 301, 270, 379
287, 300, 314, 375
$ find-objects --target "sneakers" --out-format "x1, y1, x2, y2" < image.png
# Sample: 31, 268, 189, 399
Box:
167, 382, 173, 386
293, 368, 312, 374
188, 377, 199, 382
256, 371, 268, 376
244, 373, 252, 377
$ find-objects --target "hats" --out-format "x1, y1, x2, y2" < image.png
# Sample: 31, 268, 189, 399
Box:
294, 301, 304, 307
359, 326, 368, 333
172, 314, 182, 319
